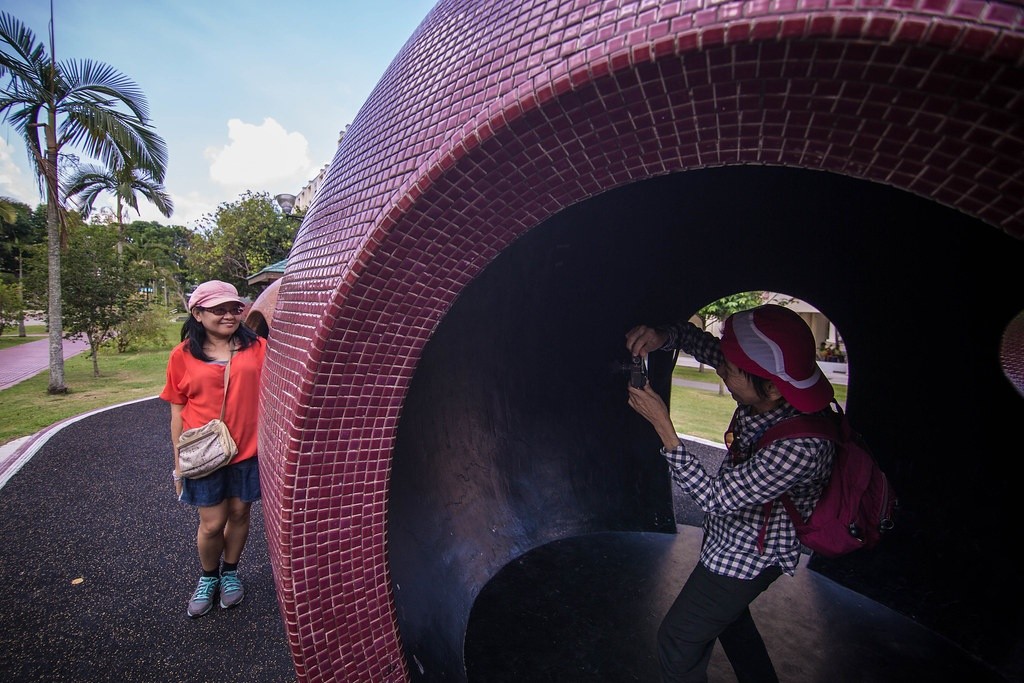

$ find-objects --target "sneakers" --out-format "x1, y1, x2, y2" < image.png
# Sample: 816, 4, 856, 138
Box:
216, 573, 244, 609
187, 576, 219, 617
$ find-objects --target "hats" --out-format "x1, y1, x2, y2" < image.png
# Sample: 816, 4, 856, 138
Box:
720, 303, 836, 412
187, 280, 246, 310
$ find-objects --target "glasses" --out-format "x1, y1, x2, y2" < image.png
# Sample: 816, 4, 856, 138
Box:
201, 307, 244, 314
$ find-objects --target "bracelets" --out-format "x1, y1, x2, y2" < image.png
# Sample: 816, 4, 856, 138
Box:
172, 469, 183, 481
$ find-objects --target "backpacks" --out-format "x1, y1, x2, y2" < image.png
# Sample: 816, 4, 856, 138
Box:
756, 400, 893, 558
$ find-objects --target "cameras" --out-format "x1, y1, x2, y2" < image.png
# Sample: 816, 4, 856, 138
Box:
612, 351, 649, 392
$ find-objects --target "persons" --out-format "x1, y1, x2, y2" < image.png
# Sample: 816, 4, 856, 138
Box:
816, 334, 847, 364
625, 304, 837, 683
248, 281, 268, 302
160, 280, 267, 618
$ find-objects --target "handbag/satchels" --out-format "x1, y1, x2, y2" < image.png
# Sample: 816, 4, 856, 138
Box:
175, 419, 238, 480
